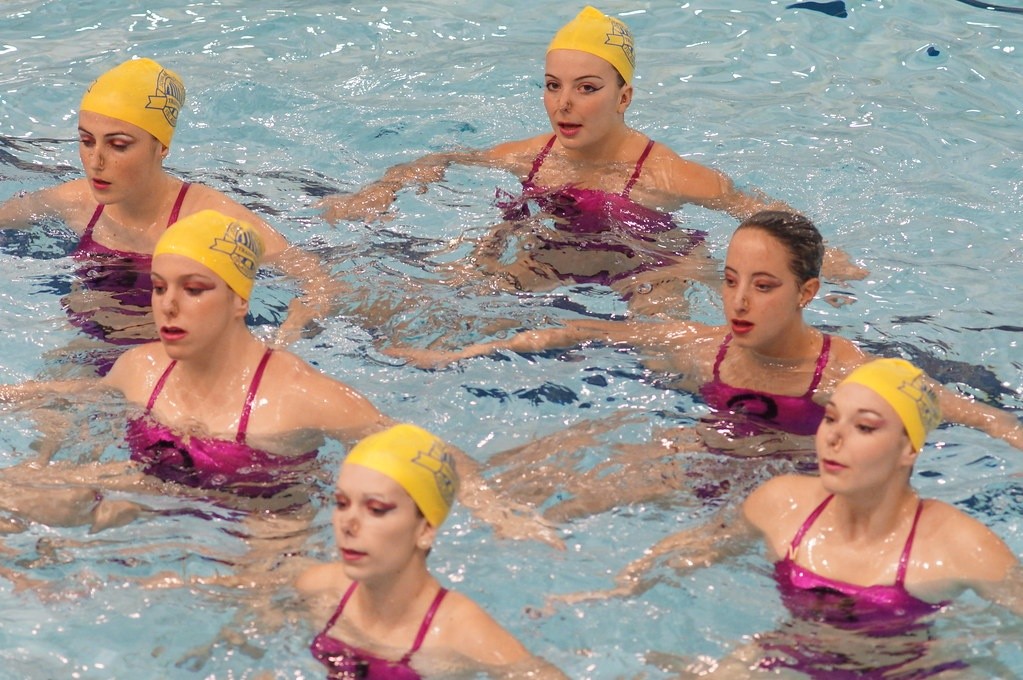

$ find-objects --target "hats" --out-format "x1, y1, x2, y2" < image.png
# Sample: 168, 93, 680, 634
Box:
346, 424, 458, 527
836, 356, 941, 454
153, 211, 265, 301
80, 58, 186, 149
544, 5, 636, 85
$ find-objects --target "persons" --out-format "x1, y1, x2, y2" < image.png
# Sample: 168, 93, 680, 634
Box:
297, 421, 528, 660
497, 5, 665, 160
744, 357, 1017, 583
105, 209, 341, 434
722, 210, 852, 361
53, 57, 285, 254
